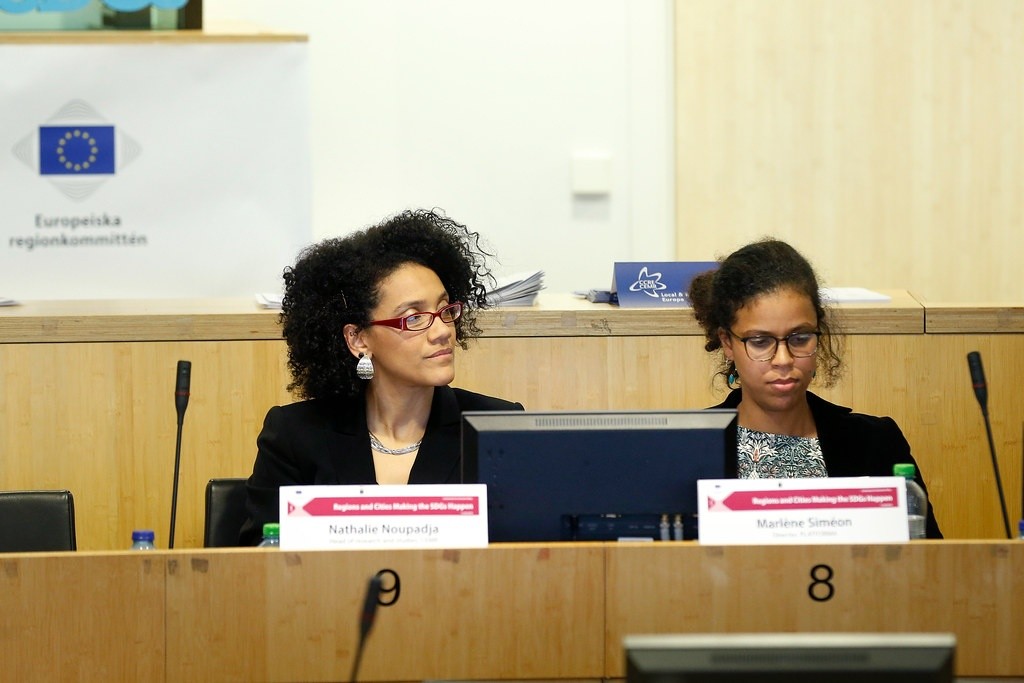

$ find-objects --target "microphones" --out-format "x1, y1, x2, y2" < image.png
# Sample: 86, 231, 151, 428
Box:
968, 351, 1013, 539
351, 577, 381, 682
168, 361, 192, 549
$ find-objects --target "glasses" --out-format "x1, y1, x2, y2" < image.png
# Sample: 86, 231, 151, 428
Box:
369, 302, 463, 331
729, 329, 822, 361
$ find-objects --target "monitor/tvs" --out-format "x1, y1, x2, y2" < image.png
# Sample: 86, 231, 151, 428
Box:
460, 408, 740, 542
625, 631, 958, 683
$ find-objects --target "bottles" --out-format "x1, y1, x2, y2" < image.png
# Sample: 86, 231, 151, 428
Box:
129, 530, 157, 550
258, 523, 279, 547
893, 463, 926, 540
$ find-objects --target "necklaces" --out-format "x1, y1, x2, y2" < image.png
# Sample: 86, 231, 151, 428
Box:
368, 429, 422, 455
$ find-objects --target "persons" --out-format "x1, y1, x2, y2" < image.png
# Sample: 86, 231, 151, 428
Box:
226, 208, 526, 547
688, 239, 942, 540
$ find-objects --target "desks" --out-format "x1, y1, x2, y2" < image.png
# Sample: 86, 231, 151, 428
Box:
0, 538, 1024, 683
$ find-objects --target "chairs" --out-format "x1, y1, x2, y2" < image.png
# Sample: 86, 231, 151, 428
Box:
0, 490, 77, 553
203, 478, 260, 547
